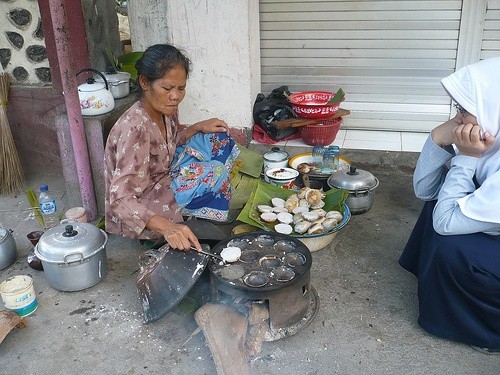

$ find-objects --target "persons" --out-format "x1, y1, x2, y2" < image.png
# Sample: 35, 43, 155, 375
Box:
104, 44, 241, 253
398, 56, 500, 354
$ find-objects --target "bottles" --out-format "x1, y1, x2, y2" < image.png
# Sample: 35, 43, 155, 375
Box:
38, 185, 59, 229
27, 231, 45, 270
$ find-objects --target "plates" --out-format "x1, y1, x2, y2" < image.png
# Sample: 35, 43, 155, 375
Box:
289, 152, 351, 180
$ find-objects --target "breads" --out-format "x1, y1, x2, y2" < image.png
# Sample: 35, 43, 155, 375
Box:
221, 247, 242, 263
257, 186, 343, 235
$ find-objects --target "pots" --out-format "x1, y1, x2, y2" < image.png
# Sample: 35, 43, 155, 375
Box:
0, 225, 16, 271
207, 231, 312, 291
34, 219, 109, 292
92, 66, 131, 99
327, 164, 379, 215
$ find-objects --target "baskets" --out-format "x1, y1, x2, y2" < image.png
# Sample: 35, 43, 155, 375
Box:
298, 116, 343, 146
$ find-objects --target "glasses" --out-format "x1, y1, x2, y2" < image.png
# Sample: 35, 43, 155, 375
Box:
453, 103, 470, 118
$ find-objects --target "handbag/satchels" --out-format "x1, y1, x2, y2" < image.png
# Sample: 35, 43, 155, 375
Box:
253, 86, 300, 140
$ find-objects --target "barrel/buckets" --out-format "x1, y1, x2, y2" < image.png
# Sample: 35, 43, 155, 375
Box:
0, 274, 39, 317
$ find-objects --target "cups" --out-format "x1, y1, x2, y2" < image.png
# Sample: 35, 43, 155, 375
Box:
263, 146, 299, 190
65, 207, 87, 223
312, 144, 339, 174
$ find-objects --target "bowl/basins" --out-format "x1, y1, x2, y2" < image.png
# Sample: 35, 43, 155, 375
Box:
259, 190, 352, 252
287, 91, 345, 119
116, 51, 144, 81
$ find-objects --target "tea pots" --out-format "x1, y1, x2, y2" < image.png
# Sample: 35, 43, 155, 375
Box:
62, 69, 115, 116
